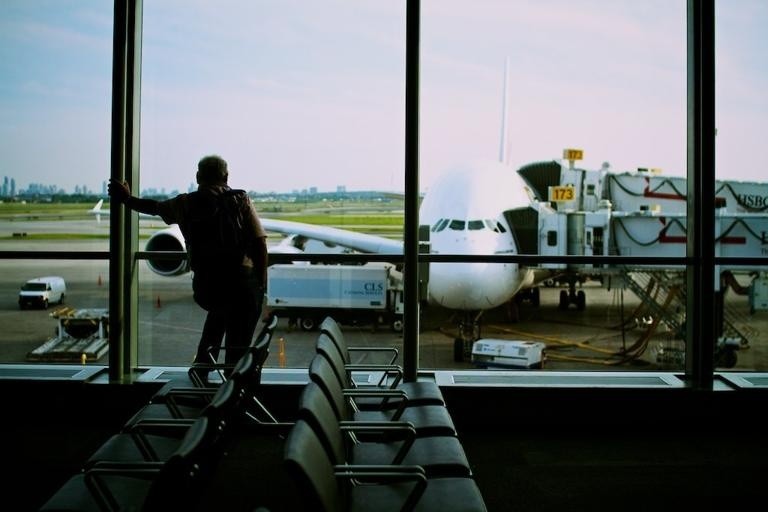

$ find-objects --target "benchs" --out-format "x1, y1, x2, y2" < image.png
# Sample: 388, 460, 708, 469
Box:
42, 315, 275, 508
285, 315, 485, 510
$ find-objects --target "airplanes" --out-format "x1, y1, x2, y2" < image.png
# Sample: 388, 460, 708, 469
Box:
87, 163, 585, 364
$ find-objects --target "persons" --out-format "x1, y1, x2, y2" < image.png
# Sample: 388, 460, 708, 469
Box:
108, 154, 269, 448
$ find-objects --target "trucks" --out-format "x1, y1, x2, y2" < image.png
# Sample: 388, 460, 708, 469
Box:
267, 261, 404, 332
26, 308, 110, 362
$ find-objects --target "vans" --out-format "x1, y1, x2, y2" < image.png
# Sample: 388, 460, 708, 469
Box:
18, 276, 66, 310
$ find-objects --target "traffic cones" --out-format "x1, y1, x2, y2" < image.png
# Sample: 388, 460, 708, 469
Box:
154, 295, 163, 309
96, 274, 104, 287
